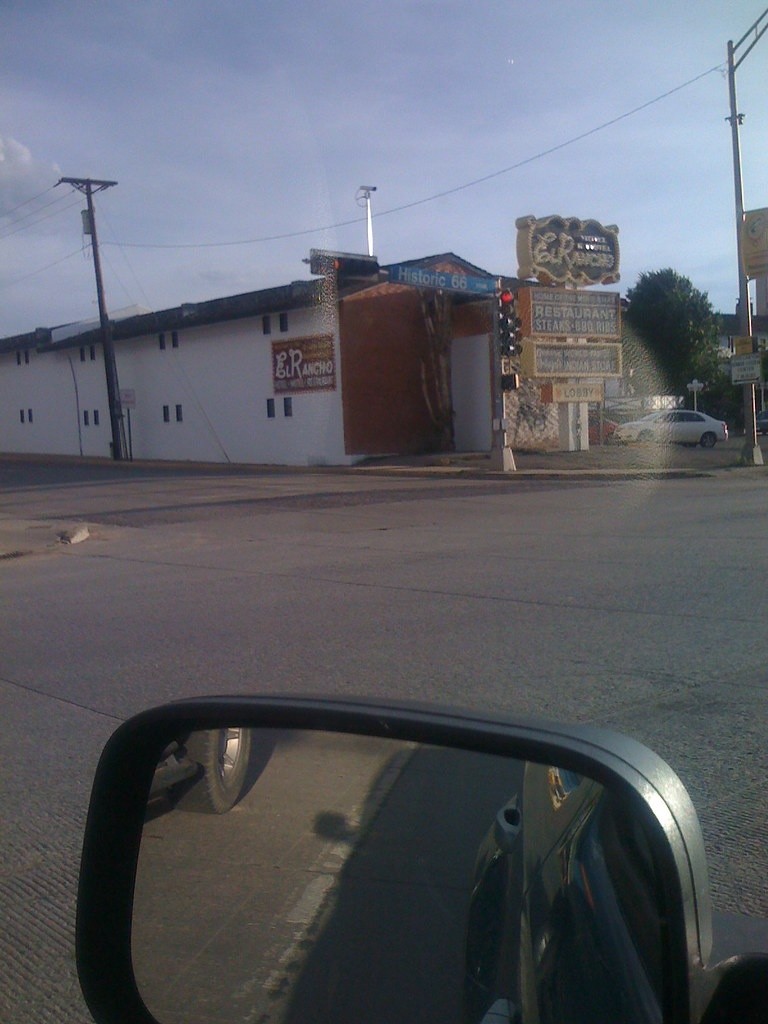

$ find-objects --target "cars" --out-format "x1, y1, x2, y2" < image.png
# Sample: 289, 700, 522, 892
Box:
755, 409, 768, 435
588, 413, 620, 446
147, 728, 252, 815
611, 409, 729, 448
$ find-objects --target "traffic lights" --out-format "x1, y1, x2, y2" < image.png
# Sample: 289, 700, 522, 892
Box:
500, 373, 519, 392
498, 288, 524, 358
